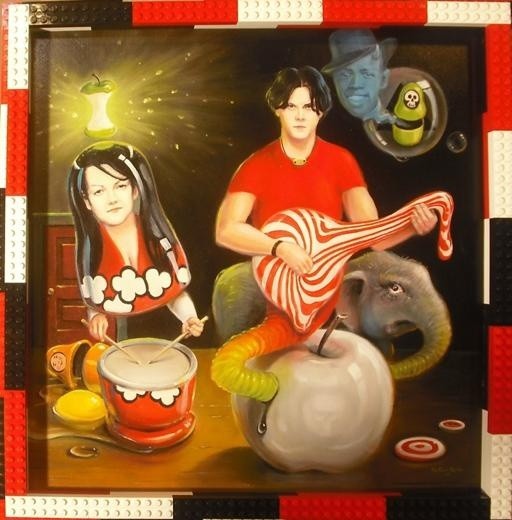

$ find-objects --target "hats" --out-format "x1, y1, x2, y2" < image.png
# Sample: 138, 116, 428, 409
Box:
320, 26, 398, 74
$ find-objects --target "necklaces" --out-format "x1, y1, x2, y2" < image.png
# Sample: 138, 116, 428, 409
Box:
280, 138, 316, 165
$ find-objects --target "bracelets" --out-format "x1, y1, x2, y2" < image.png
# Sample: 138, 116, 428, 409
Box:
272, 240, 282, 258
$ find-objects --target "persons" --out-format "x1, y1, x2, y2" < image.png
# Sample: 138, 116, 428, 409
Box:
65, 142, 204, 342
315, 28, 399, 121
209, 64, 438, 338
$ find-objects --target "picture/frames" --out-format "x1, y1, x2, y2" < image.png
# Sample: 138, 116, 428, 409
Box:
2, 0, 512, 520
